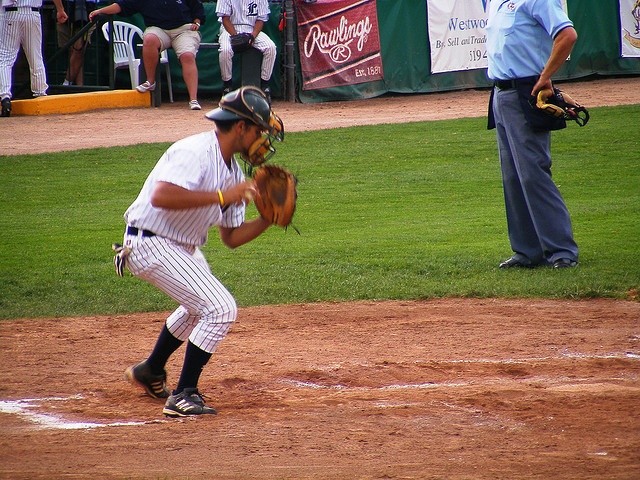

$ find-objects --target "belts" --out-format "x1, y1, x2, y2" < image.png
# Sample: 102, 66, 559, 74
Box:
495, 74, 539, 90
4, 6, 40, 12
126, 225, 156, 238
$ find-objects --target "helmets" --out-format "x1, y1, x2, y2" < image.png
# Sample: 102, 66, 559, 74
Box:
205, 85, 286, 166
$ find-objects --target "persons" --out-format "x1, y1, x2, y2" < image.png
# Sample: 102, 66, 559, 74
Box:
110, 86, 300, 417
53, 0, 98, 86
486, 0, 590, 268
0, 0, 49, 117
87, 0, 204, 109
214, 0, 278, 95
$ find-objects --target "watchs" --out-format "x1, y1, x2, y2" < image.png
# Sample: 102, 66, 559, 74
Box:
194, 21, 200, 27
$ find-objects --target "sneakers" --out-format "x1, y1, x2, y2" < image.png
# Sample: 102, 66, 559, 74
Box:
1, 98, 13, 117
137, 81, 157, 93
189, 100, 202, 109
162, 388, 217, 417
126, 359, 169, 400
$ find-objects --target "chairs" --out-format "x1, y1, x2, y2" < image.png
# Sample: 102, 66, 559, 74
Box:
101, 21, 168, 103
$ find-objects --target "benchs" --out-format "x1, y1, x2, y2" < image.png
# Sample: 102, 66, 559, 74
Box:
137, 43, 277, 103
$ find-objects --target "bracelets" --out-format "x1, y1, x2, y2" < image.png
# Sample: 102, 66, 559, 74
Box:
217, 189, 225, 208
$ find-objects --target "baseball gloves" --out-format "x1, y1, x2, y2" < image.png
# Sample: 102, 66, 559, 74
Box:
230, 32, 253, 53
252, 165, 301, 236
536, 86, 588, 127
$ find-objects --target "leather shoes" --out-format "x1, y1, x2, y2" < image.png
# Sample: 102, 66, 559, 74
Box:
500, 258, 537, 269
554, 259, 579, 268
